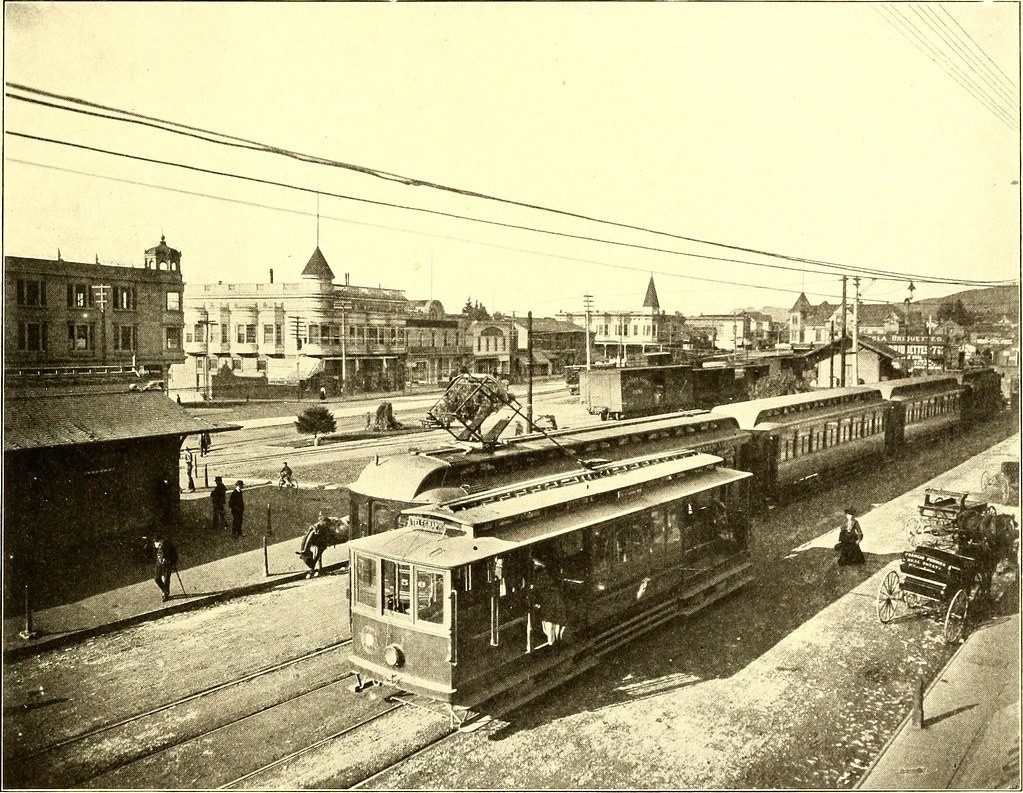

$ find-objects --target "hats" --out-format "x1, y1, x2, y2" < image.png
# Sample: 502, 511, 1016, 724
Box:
844, 509, 854, 515
214, 476, 223, 481
234, 481, 245, 485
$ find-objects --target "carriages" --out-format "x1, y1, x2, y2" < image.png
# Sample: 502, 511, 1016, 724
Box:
876, 460, 1020, 645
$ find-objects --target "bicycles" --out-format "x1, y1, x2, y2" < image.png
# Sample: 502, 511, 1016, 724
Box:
277, 473, 299, 496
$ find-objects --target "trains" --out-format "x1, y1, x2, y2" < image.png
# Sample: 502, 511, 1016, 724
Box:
344, 329, 1003, 580
579, 369, 806, 420
344, 368, 757, 735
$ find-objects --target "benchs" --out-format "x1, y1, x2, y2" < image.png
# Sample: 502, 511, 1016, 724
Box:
1001, 462, 1018, 491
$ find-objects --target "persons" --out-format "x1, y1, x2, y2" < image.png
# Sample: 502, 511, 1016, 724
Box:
184, 450, 194, 493
833, 507, 863, 565
320, 383, 326, 400
211, 477, 227, 528
229, 480, 245, 539
177, 394, 182, 404
280, 462, 294, 486
151, 532, 178, 602
198, 432, 211, 457
